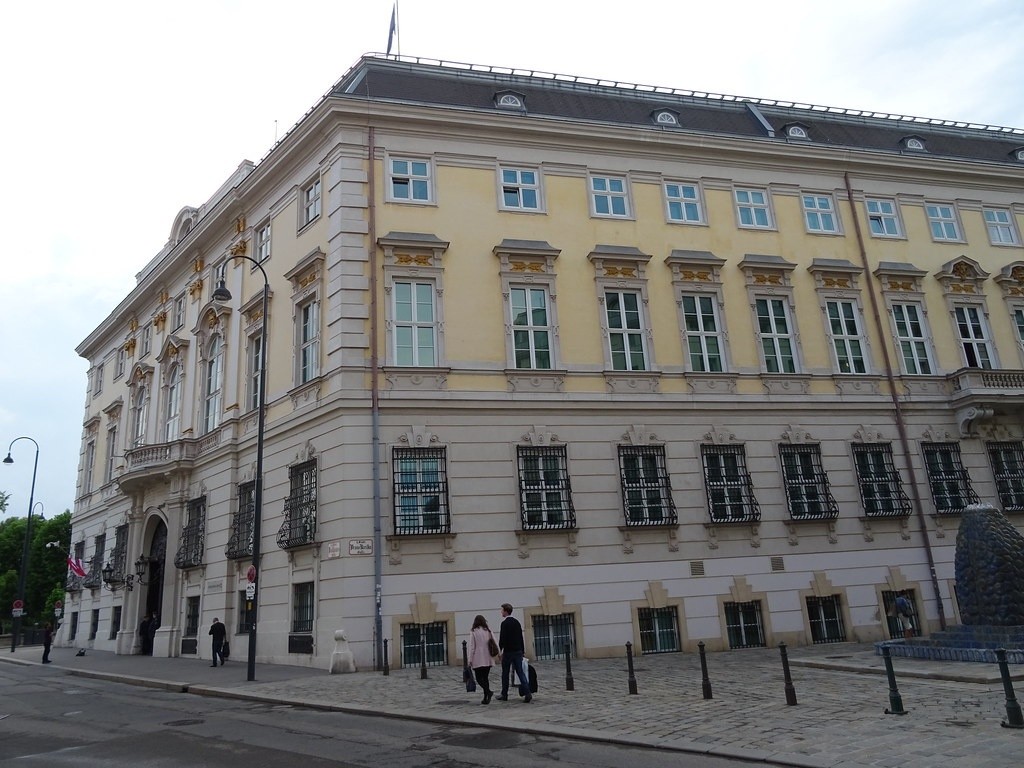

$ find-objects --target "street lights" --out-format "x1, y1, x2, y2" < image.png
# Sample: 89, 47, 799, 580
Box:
211, 254, 270, 681
26, 500, 45, 617
4, 435, 39, 652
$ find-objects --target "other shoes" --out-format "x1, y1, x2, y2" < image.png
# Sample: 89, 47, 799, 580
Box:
482, 691, 493, 704
211, 665, 217, 667
524, 694, 532, 703
496, 695, 507, 701
221, 658, 224, 665
42, 660, 51, 664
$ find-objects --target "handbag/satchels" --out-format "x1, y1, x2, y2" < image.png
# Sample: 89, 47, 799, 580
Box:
222, 640, 230, 657
519, 665, 537, 696
514, 657, 530, 684
466, 668, 476, 691
488, 632, 499, 656
904, 608, 914, 618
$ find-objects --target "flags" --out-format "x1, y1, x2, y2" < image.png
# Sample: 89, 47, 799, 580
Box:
68, 554, 87, 578
386, 4, 396, 58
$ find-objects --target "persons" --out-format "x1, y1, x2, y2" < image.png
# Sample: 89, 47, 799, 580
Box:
139, 611, 159, 656
495, 603, 532, 703
208, 618, 226, 667
469, 614, 501, 705
43, 621, 56, 664
895, 590, 913, 639
